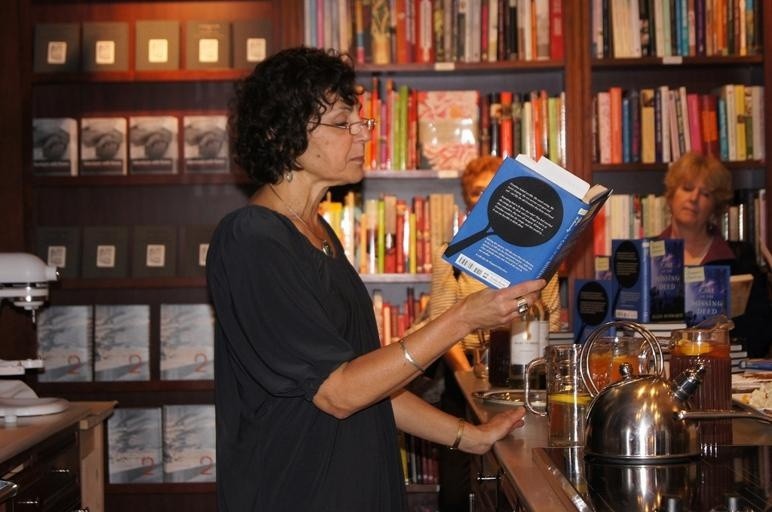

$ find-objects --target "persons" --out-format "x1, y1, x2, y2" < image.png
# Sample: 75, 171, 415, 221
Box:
204, 41, 548, 510
427, 151, 564, 511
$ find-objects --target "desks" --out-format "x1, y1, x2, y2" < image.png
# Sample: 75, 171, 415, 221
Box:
0, 396, 123, 512
450, 357, 772, 512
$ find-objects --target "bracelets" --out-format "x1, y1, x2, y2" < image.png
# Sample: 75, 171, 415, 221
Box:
449, 415, 466, 452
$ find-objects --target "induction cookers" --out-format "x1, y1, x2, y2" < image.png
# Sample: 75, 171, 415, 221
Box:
527, 443, 771, 512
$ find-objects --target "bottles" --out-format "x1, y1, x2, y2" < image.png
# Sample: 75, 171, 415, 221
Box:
486, 291, 550, 389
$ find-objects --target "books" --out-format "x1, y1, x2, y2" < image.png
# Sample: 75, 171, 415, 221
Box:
394, 423, 442, 490
30, 20, 287, 71
34, 305, 215, 382
41, 403, 220, 488
31, 225, 214, 279
303, 2, 568, 348
588, 1, 771, 387
108, 403, 217, 484
32, 111, 231, 175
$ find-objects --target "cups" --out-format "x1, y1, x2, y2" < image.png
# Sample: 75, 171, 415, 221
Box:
668, 328, 732, 414
587, 336, 652, 396
473, 346, 489, 380
525, 343, 588, 446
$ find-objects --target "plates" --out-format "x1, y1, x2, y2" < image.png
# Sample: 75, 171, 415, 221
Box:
469, 388, 546, 408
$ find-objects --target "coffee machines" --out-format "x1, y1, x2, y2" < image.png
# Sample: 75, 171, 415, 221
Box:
2, 251, 70, 417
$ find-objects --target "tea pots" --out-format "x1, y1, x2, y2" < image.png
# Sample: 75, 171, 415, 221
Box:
582, 456, 702, 511
579, 318, 712, 464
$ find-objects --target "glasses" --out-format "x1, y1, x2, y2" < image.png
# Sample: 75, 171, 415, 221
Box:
309, 117, 377, 135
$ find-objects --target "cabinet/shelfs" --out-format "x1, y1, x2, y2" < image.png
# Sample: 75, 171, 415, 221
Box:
281, 0, 771, 495
21, 65, 266, 495
